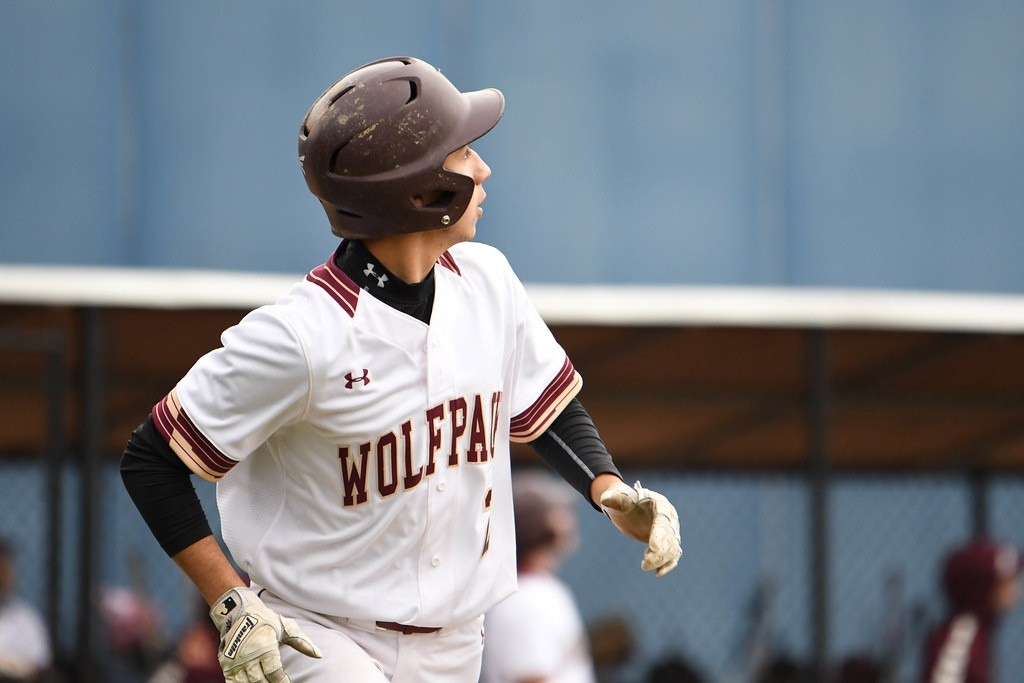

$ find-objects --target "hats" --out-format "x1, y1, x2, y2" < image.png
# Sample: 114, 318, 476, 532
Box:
946, 545, 1023, 600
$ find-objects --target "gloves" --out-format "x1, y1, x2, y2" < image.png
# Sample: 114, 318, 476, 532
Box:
600, 480, 682, 576
210, 587, 323, 683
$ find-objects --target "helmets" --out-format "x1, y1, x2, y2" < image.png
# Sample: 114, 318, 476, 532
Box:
511, 471, 576, 549
299, 54, 506, 239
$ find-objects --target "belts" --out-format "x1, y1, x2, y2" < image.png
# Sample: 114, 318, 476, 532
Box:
376, 619, 442, 636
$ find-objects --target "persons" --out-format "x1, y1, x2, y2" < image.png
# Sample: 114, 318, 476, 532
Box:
920, 540, 1024, 683
481, 468, 595, 683
120, 57, 683, 683
0, 535, 250, 683
